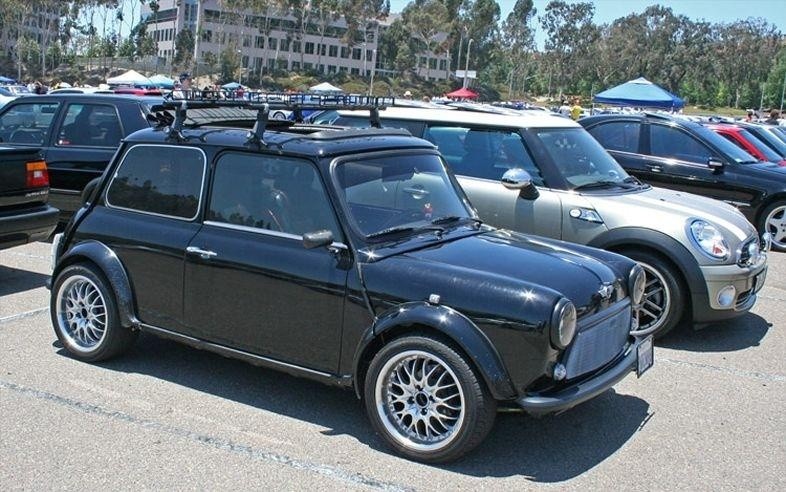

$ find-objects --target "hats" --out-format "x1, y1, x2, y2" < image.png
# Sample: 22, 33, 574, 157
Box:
179, 71, 195, 80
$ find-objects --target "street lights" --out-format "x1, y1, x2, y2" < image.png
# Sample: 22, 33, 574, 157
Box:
461, 39, 474, 103
235, 47, 243, 85
369, 48, 377, 97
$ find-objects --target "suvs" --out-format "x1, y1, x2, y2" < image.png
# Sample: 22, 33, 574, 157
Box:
293, 107, 768, 343
44, 89, 655, 464
